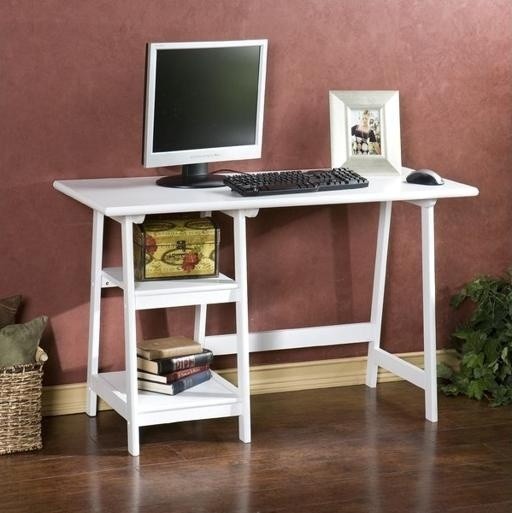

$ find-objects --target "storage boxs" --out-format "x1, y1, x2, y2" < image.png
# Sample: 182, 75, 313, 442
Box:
132, 219, 222, 281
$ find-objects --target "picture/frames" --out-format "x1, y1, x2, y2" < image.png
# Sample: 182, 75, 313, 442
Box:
328, 89, 402, 180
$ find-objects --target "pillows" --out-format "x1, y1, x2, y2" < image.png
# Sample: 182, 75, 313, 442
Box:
0, 315, 49, 370
0, 293, 23, 331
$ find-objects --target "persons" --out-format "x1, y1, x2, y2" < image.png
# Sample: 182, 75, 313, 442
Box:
351, 109, 381, 155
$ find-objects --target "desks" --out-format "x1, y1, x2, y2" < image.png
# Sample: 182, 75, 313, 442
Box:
52, 166, 480, 456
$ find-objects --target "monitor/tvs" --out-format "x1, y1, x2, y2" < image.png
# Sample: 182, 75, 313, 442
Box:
141, 39, 269, 189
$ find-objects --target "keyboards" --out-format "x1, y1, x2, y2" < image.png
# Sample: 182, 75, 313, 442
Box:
222, 167, 369, 197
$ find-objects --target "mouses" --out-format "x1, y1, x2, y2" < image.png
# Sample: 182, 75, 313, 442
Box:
406, 168, 444, 185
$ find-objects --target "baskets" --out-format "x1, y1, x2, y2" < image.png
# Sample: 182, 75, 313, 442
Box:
0, 361, 44, 455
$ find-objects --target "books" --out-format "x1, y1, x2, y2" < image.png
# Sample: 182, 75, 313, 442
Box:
137, 334, 214, 395
